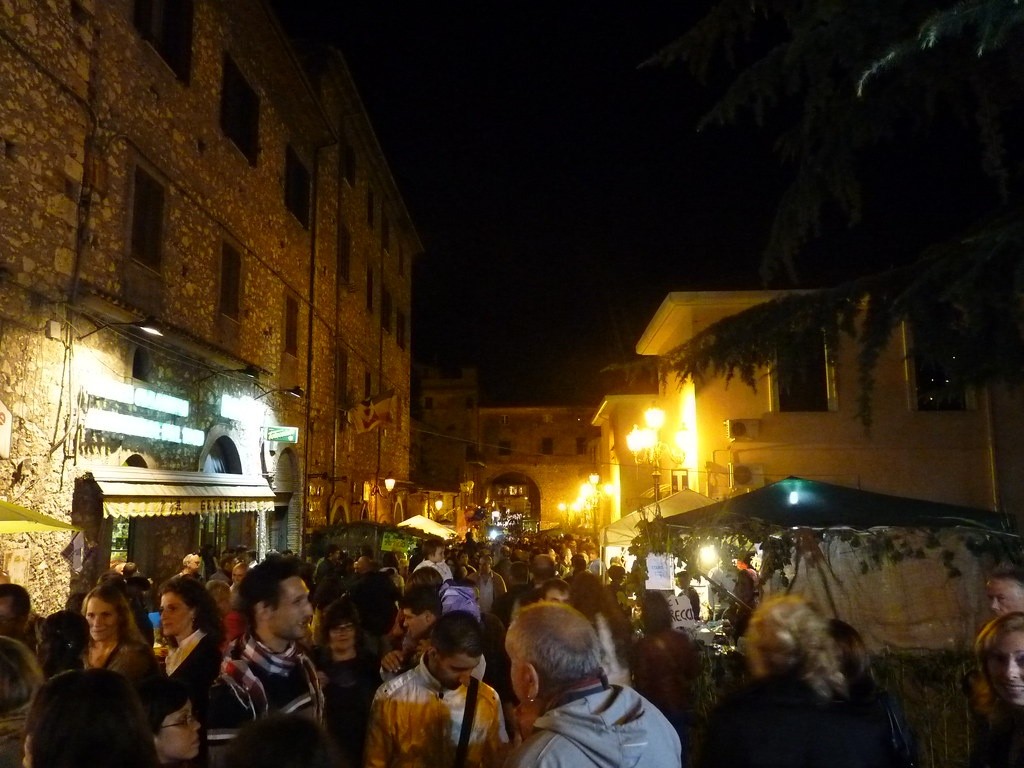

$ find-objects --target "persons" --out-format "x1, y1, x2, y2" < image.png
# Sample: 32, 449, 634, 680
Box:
0, 523, 1024, 768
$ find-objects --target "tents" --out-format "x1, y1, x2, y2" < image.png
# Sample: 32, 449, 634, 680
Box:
659, 475, 1023, 656
598, 487, 721, 588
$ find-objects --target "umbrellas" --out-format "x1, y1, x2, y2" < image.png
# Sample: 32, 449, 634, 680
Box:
0, 501, 84, 535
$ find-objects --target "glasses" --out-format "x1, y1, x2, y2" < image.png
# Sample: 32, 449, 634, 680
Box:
333, 624, 354, 634
162, 711, 199, 728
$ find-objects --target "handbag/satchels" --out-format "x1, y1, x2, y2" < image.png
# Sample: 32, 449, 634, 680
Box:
886, 706, 919, 768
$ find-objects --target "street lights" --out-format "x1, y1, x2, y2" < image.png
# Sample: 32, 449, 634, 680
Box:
625, 406, 697, 519
362, 473, 396, 557
420, 490, 443, 521
571, 471, 615, 536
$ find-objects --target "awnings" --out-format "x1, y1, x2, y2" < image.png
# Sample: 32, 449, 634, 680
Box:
393, 514, 457, 541
85, 470, 276, 520
